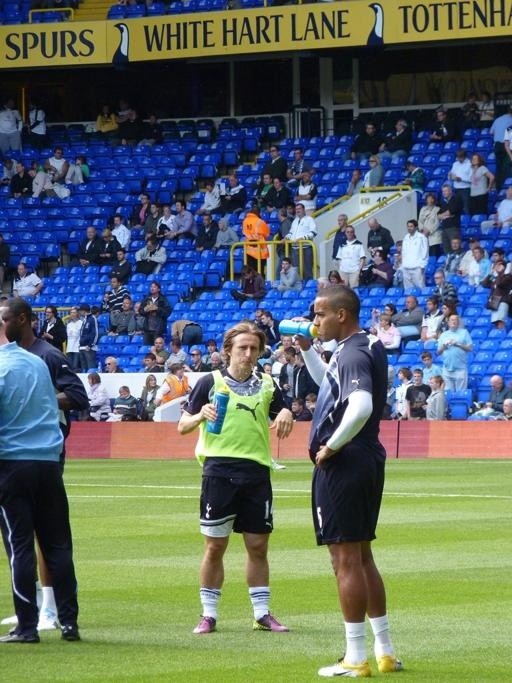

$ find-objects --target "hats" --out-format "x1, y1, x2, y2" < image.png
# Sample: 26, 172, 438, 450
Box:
77, 303, 90, 311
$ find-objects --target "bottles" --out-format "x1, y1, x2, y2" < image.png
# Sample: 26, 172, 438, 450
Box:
278, 318, 320, 339
205, 387, 230, 436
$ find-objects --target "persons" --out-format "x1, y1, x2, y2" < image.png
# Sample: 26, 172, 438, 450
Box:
1, 92, 511, 423
0, 295, 90, 631
0, 321, 81, 644
292, 284, 403, 678
175, 320, 294, 635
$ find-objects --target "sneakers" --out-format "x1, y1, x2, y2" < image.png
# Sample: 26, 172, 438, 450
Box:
376, 655, 403, 674
252, 613, 290, 635
0, 624, 40, 645
57, 622, 83, 643
193, 615, 217, 635
315, 655, 373, 681
0, 612, 61, 632
